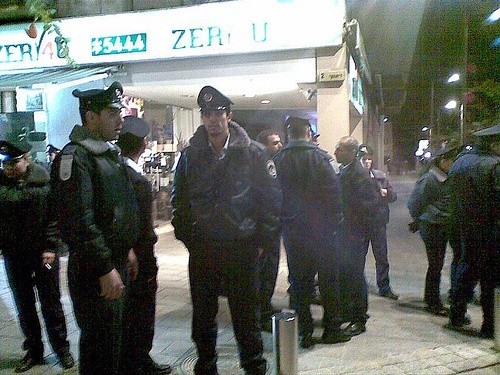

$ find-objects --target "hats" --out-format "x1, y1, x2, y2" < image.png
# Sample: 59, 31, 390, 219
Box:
282, 114, 314, 135
72, 80, 128, 109
356, 144, 374, 159
197, 85, 234, 112
45, 144, 61, 153
119, 115, 151, 143
0, 139, 33, 160
473, 125, 500, 140
432, 146, 456, 161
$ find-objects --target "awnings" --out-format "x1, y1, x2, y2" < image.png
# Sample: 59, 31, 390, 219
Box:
0, 65, 121, 93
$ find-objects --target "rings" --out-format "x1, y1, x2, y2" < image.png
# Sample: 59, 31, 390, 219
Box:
120, 285, 125, 289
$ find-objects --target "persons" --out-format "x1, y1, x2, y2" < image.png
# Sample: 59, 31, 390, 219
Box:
51, 80, 138, 375
444, 122, 500, 337
111, 115, 172, 375
331, 136, 378, 336
171, 85, 283, 375
0, 137, 74, 372
406, 144, 458, 317
252, 111, 351, 348
354, 144, 400, 300
44, 143, 70, 257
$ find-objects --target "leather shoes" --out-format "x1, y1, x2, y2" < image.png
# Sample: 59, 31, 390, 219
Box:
56, 346, 74, 368
16, 348, 43, 373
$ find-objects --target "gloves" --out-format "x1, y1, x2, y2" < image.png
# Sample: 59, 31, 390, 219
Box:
408, 219, 421, 234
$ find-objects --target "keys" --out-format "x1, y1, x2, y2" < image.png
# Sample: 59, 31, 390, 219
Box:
45, 262, 52, 270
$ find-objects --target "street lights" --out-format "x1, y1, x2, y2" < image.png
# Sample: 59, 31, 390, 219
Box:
430, 74, 459, 130
437, 100, 457, 134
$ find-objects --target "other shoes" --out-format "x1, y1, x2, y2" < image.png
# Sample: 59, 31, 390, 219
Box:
262, 318, 272, 332
344, 322, 366, 336
144, 361, 171, 375
379, 286, 398, 299
426, 303, 451, 316
467, 291, 481, 306
310, 290, 322, 305
301, 333, 317, 349
320, 327, 351, 344
449, 312, 471, 325
